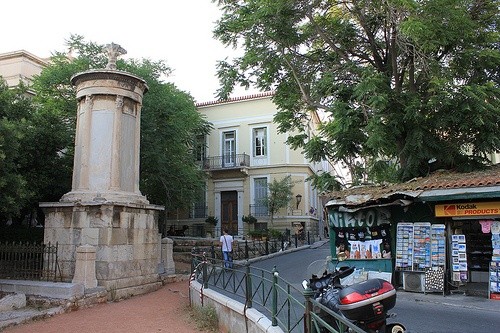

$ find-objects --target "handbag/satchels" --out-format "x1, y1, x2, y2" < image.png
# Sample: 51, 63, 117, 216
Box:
228, 252, 233, 260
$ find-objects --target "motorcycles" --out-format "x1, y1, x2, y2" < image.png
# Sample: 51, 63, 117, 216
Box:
302, 252, 406, 333
167, 224, 190, 237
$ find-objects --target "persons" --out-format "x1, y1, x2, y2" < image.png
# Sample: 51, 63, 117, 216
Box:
219, 228, 234, 272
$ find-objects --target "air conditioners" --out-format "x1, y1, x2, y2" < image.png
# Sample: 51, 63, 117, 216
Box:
402, 272, 435, 293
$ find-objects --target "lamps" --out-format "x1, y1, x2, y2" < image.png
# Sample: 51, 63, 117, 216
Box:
295, 193, 302, 209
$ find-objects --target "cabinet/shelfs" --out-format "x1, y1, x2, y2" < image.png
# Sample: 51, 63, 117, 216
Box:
448, 231, 500, 300
394, 222, 447, 273
